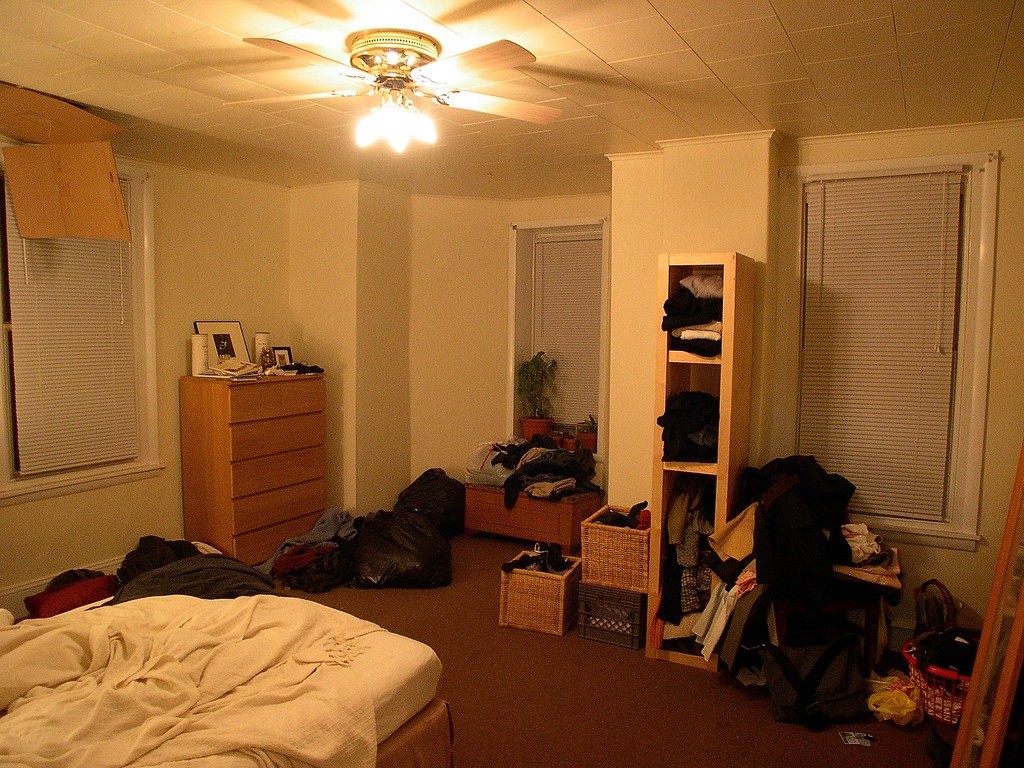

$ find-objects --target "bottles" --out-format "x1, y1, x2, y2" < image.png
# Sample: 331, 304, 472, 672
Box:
263, 347, 274, 368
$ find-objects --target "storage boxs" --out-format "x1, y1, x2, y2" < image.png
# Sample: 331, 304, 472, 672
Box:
578, 580, 648, 650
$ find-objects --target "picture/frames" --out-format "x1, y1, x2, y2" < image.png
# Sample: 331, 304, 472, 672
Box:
194, 321, 251, 368
272, 346, 293, 366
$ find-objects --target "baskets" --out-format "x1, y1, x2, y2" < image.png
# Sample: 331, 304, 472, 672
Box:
498, 547, 588, 637
902, 627, 980, 729
575, 582, 645, 652
578, 503, 663, 595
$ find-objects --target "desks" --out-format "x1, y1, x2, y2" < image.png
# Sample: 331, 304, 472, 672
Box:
774, 600, 878, 665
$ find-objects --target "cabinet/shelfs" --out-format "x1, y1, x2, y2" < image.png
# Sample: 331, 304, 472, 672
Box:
179, 373, 328, 566
645, 249, 757, 672
464, 481, 601, 557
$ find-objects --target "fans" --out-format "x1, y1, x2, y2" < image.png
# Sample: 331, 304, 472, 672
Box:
222, 29, 563, 126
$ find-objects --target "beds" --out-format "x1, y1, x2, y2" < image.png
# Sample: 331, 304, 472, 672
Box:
0, 594, 455, 768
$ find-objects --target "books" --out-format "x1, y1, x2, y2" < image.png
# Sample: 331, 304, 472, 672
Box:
193, 358, 265, 381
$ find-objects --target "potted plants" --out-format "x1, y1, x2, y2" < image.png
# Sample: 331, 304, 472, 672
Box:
518, 351, 598, 452
